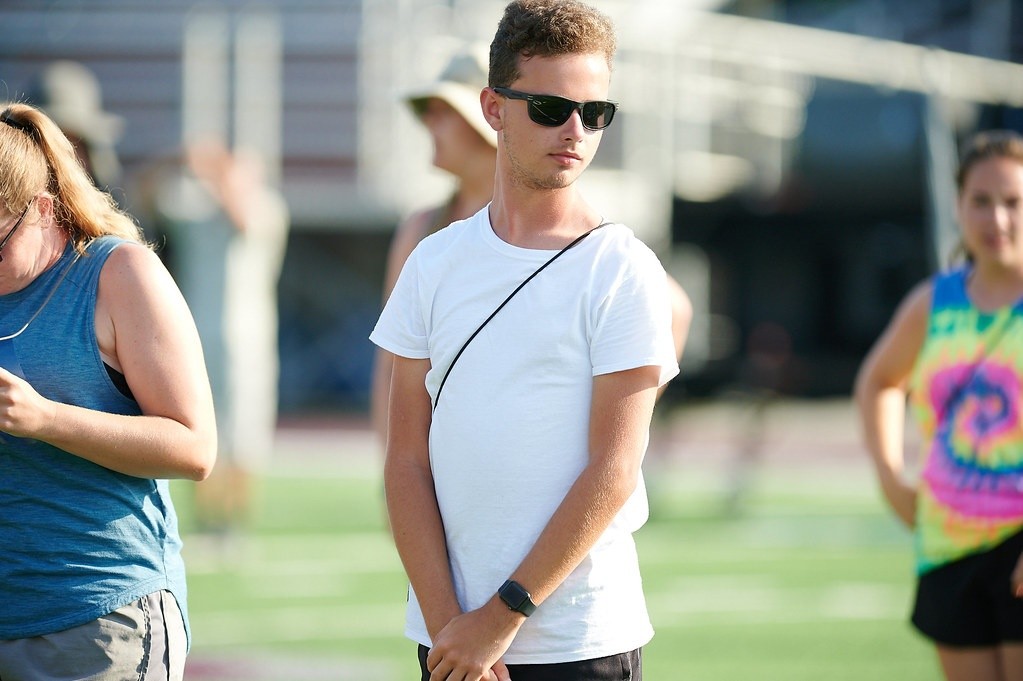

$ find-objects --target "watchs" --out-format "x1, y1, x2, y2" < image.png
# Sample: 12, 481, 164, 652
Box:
497, 579, 538, 618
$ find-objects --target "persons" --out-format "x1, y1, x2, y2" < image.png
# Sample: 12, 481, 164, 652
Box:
369, 3, 681, 681
17, 57, 291, 537
0, 103, 219, 681
852, 131, 1023, 681
367, 48, 694, 450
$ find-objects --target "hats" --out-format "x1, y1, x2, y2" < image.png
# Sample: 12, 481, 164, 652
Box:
406, 50, 499, 148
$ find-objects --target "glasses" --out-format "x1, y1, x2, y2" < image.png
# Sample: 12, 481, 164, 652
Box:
495, 84, 614, 130
